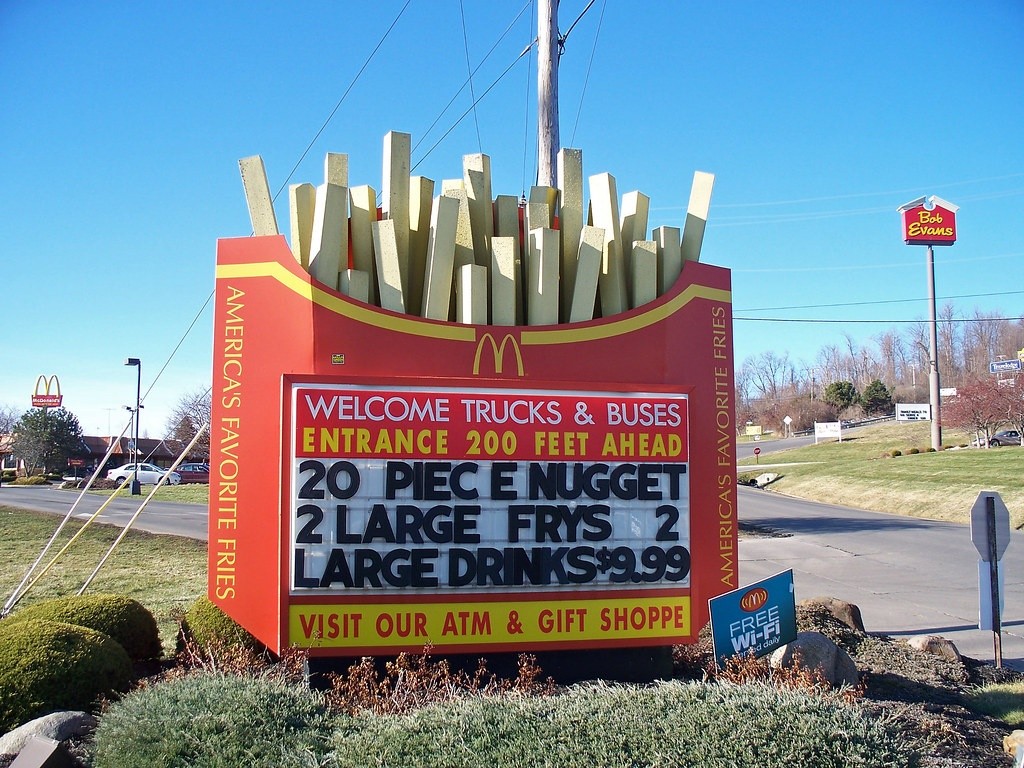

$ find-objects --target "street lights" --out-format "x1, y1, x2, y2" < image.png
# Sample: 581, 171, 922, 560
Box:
124, 356, 141, 495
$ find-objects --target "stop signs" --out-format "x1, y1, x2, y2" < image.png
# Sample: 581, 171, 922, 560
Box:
754, 447, 760, 454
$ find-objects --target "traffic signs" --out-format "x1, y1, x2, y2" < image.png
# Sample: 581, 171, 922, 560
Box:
68, 457, 86, 467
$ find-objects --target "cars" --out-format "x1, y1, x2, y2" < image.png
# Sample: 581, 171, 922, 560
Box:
175, 462, 209, 484
163, 467, 170, 471
840, 413, 896, 429
107, 461, 181, 485
972, 431, 1024, 447
